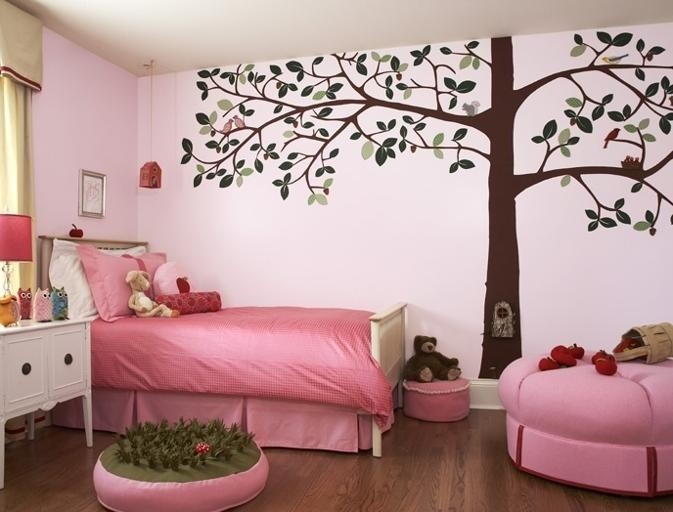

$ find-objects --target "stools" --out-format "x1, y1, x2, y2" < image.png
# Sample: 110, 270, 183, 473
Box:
496, 352, 673, 497
401, 377, 471, 424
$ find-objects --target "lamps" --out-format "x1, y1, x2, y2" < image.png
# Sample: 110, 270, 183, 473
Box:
0, 214, 33, 291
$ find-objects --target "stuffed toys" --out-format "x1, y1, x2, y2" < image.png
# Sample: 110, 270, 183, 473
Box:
49, 286, 69, 320
400, 334, 462, 383
124, 270, 180, 317
16, 287, 32, 320
33, 288, 52, 322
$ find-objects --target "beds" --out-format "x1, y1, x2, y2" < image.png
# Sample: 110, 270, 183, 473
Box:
38, 234, 409, 458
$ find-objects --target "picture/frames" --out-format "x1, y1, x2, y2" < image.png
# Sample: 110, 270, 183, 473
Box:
78, 167, 106, 219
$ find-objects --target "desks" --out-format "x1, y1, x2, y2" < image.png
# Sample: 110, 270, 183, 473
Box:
0, 316, 95, 489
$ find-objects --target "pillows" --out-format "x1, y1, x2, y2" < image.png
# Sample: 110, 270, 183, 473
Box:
150, 291, 221, 314
76, 243, 166, 322
49, 238, 147, 321
154, 261, 200, 294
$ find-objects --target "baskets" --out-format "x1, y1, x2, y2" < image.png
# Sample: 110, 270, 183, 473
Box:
613, 322, 673, 364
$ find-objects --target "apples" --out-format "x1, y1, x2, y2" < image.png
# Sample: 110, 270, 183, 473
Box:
69, 224, 83, 238
538, 344, 617, 375
